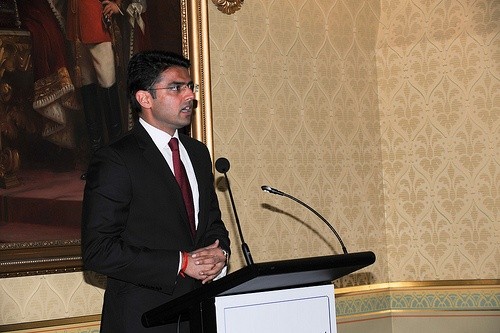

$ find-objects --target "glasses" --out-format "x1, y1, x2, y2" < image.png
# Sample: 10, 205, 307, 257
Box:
147, 84, 194, 96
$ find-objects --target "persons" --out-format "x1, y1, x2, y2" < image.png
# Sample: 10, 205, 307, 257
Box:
81, 47, 232, 333
18, 0, 145, 181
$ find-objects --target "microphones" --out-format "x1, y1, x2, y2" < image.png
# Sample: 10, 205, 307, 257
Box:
261, 185, 348, 254
214, 157, 254, 266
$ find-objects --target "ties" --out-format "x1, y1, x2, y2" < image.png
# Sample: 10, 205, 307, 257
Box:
168, 138, 195, 237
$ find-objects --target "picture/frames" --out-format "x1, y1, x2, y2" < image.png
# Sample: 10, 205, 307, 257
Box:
0, 0, 215, 279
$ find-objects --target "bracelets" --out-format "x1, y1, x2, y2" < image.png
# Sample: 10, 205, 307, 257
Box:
180, 251, 188, 279
